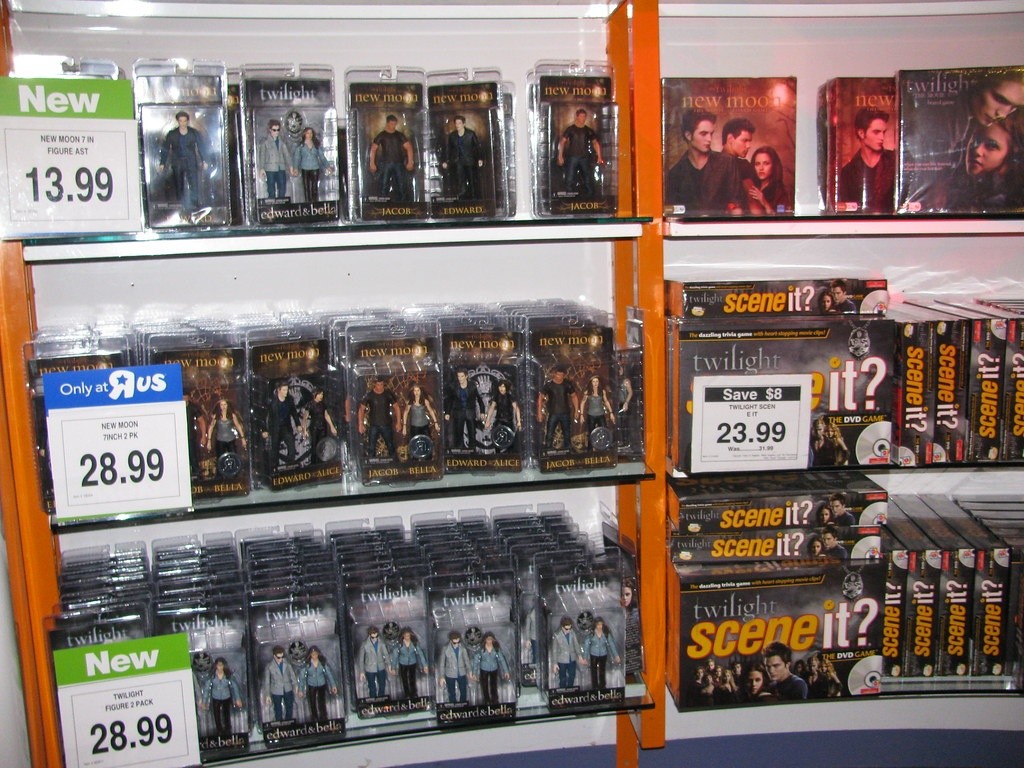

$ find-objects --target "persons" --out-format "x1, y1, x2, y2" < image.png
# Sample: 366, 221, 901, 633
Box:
192, 616, 622, 735
811, 417, 847, 464
806, 498, 855, 557
188, 366, 632, 475
689, 642, 840, 702
664, 74, 1022, 215
816, 279, 855, 316
159, 107, 606, 219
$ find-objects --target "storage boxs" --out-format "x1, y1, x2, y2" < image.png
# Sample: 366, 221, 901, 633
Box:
18, 56, 644, 768
663, 66, 1024, 709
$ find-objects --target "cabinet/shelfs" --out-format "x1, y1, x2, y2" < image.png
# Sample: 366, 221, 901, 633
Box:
0, 1, 656, 767
627, 3, 1024, 749
